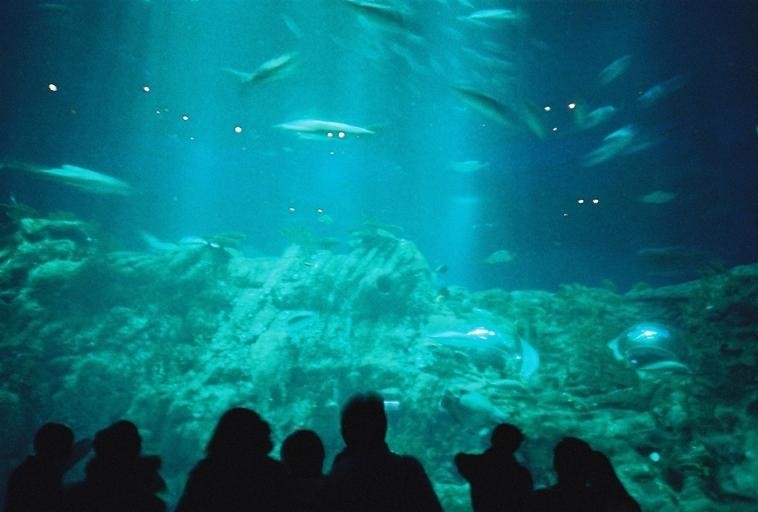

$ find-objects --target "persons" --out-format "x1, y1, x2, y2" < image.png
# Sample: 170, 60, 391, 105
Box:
5, 420, 173, 511
176, 391, 444, 512
455, 424, 641, 511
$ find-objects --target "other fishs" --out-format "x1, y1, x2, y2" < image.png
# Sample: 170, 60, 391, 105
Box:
330, 0, 674, 170
